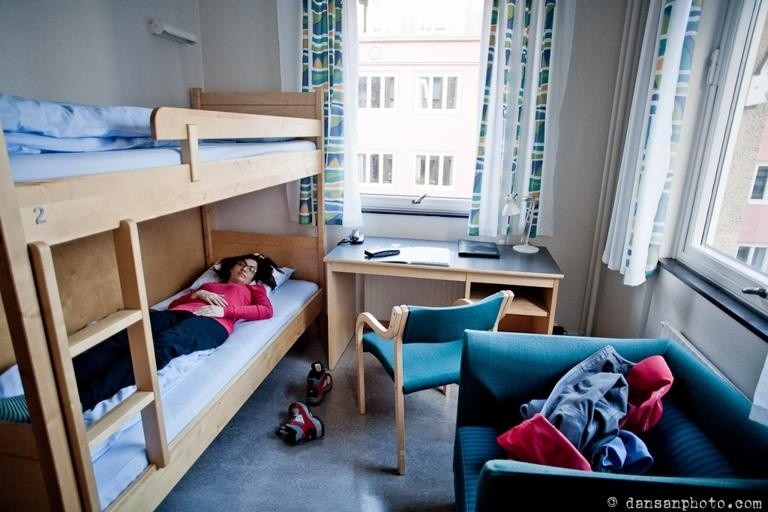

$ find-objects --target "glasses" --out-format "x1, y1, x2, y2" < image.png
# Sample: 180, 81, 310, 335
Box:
237, 261, 256, 272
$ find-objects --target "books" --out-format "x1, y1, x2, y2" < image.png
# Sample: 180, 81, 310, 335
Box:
372, 244, 451, 268
458, 239, 500, 259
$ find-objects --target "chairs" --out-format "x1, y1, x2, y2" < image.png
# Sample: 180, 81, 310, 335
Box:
355, 289, 515, 475
454, 329, 768, 512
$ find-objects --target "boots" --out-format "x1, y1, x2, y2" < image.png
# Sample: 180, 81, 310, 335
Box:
306, 360, 333, 406
278, 401, 325, 445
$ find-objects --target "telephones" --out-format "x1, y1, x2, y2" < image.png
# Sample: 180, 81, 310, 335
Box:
349, 228, 364, 244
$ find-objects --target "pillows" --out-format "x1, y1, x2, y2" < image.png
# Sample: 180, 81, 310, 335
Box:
190, 259, 296, 299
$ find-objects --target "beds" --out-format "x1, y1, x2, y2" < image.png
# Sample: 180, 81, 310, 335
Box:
0, 87, 336, 512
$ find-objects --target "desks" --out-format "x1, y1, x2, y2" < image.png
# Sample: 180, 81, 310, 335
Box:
323, 234, 565, 371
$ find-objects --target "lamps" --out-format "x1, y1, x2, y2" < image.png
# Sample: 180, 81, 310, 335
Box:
502, 192, 540, 254
148, 19, 198, 48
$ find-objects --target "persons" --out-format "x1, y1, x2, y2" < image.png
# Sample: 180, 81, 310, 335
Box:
1, 247, 289, 438
